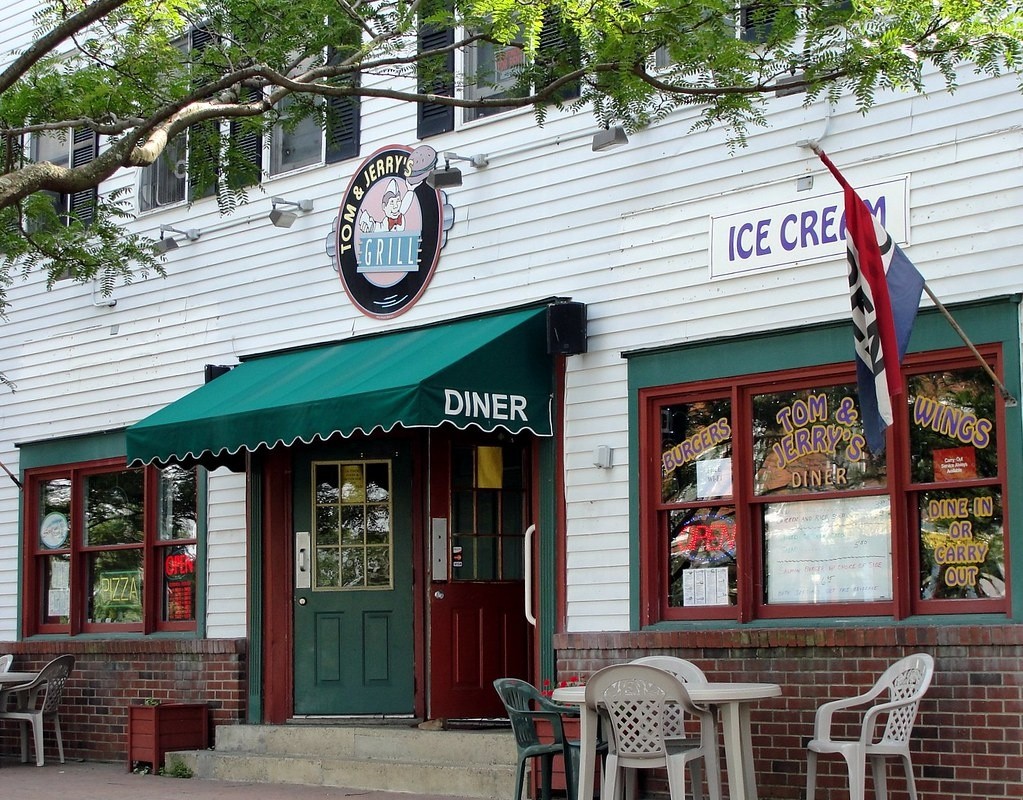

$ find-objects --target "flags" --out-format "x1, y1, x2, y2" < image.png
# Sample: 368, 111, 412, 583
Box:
841, 185, 925, 451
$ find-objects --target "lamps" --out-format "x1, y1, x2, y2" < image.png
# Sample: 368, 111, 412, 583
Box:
592, 111, 650, 153
268, 196, 313, 228
775, 73, 839, 99
426, 151, 488, 189
151, 223, 199, 257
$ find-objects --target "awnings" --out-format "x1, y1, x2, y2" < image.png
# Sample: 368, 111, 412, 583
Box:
125, 304, 554, 468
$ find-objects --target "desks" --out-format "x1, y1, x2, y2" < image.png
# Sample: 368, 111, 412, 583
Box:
551, 683, 782, 800
0, 671, 39, 685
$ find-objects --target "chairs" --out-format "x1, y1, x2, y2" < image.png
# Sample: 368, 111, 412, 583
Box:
0, 654, 75, 767
493, 677, 608, 800
806, 653, 934, 800
0, 654, 15, 691
584, 663, 723, 800
624, 655, 708, 800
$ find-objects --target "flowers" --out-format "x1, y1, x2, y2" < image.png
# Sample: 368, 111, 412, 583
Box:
540, 676, 587, 718
